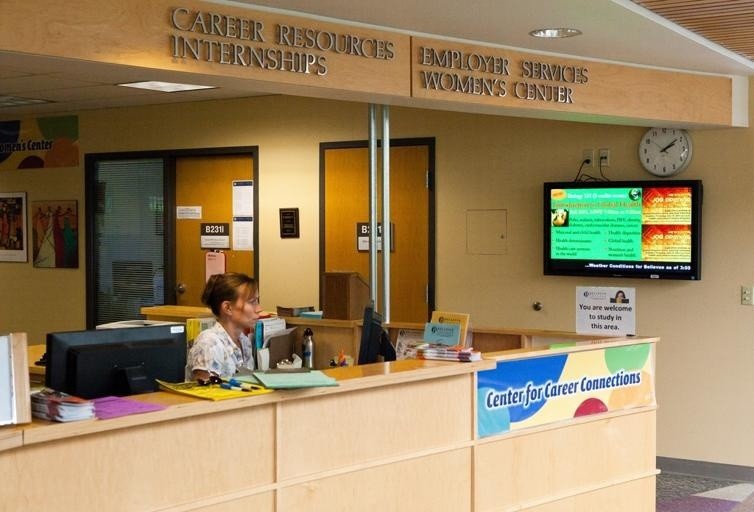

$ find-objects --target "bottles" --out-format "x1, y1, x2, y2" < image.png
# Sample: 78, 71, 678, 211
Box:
302, 328, 315, 369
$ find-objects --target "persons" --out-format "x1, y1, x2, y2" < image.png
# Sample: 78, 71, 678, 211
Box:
185, 272, 265, 383
615, 291, 625, 298
552, 209, 569, 227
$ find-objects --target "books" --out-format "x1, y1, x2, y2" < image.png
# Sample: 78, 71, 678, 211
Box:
395, 311, 482, 362
0, 336, 13, 426
186, 317, 216, 349
31, 387, 96, 422
9, 332, 32, 424
247, 305, 323, 369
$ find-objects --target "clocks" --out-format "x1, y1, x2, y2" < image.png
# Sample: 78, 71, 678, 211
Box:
637, 127, 693, 178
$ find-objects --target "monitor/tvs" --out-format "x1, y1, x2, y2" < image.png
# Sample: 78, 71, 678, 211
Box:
358, 300, 396, 364
541, 179, 704, 281
45, 323, 188, 400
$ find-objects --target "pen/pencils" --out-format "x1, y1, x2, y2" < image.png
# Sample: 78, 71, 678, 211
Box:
330, 349, 348, 368
221, 379, 261, 392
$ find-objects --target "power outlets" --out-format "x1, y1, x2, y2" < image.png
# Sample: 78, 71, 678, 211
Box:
599, 148, 610, 168
582, 150, 594, 169
